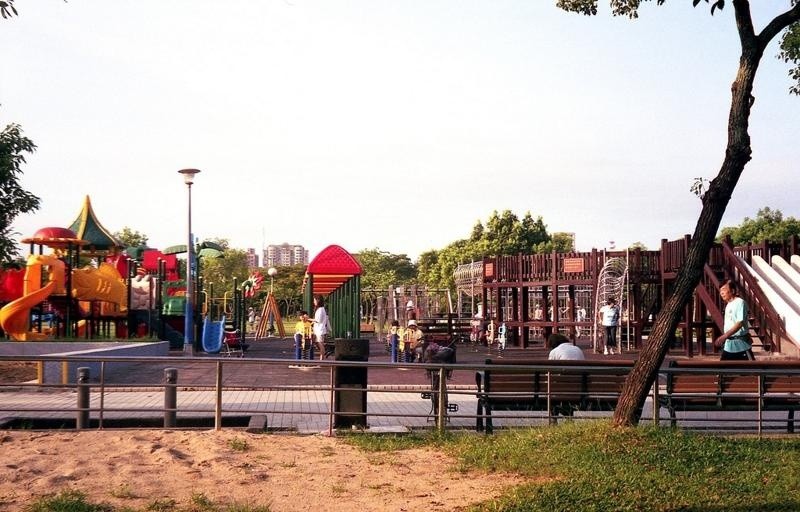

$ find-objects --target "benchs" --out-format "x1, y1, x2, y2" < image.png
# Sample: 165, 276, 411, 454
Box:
360, 324, 375, 336
652, 359, 799, 434
474, 359, 638, 435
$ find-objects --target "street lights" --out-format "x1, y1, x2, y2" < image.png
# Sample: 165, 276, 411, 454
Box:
267, 268, 277, 338
177, 168, 202, 356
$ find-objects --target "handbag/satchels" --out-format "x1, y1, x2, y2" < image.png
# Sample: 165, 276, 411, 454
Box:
744, 333, 753, 344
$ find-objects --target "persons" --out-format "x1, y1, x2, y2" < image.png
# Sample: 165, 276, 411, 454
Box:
407, 319, 425, 362
548, 334, 585, 424
599, 299, 619, 355
249, 307, 255, 330
534, 303, 587, 339
293, 311, 311, 350
311, 294, 327, 354
714, 280, 756, 360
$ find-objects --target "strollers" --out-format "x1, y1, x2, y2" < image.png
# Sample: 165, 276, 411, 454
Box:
220, 327, 245, 359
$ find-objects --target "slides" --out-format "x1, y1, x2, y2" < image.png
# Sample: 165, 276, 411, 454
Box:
0, 254, 66, 343
201, 316, 226, 354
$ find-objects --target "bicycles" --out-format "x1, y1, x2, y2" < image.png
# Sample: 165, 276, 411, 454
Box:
416, 337, 459, 424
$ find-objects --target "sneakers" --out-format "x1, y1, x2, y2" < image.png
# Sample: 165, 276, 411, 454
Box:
610, 348, 614, 355
604, 348, 608, 355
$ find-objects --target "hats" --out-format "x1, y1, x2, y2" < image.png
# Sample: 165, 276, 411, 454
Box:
408, 320, 417, 326
